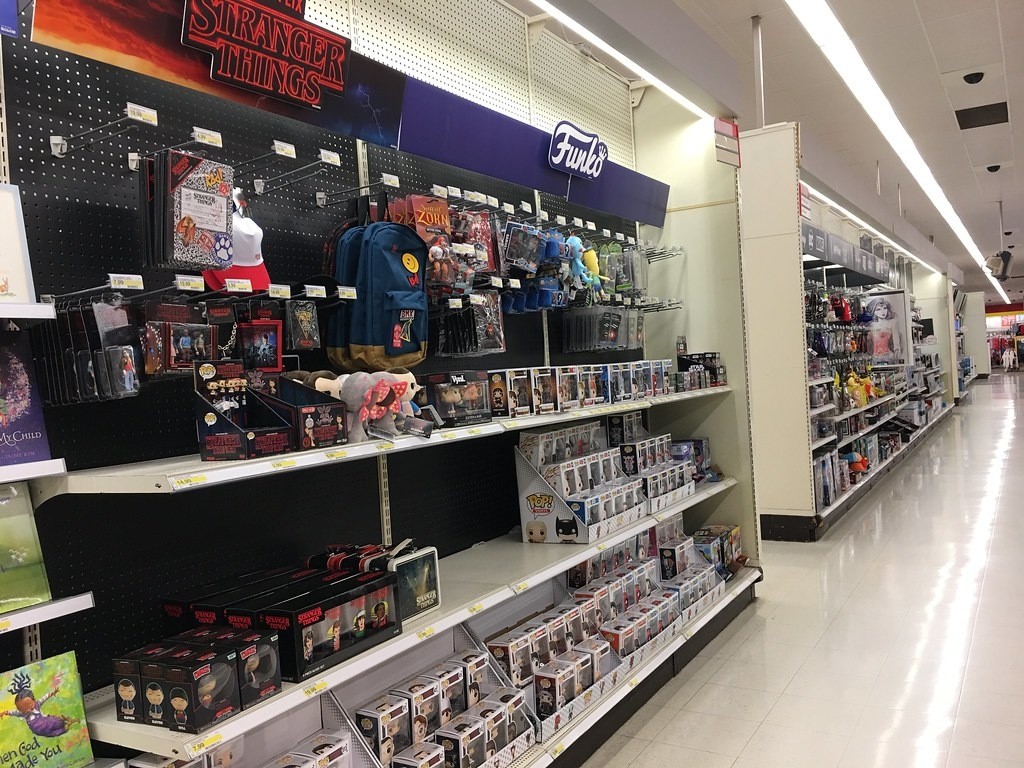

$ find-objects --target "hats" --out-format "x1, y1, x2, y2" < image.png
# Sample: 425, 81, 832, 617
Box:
201, 211, 272, 294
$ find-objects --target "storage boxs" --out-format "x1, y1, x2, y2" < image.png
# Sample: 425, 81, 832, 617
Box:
955, 290, 976, 391
802, 193, 946, 514
0, 181, 741, 768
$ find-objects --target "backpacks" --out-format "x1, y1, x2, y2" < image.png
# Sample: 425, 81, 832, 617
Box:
322, 189, 428, 372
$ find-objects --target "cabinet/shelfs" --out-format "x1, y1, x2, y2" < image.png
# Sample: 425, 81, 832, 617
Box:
948, 277, 979, 405
737, 120, 956, 542
987, 330, 1024, 372
0, 0, 764, 768
